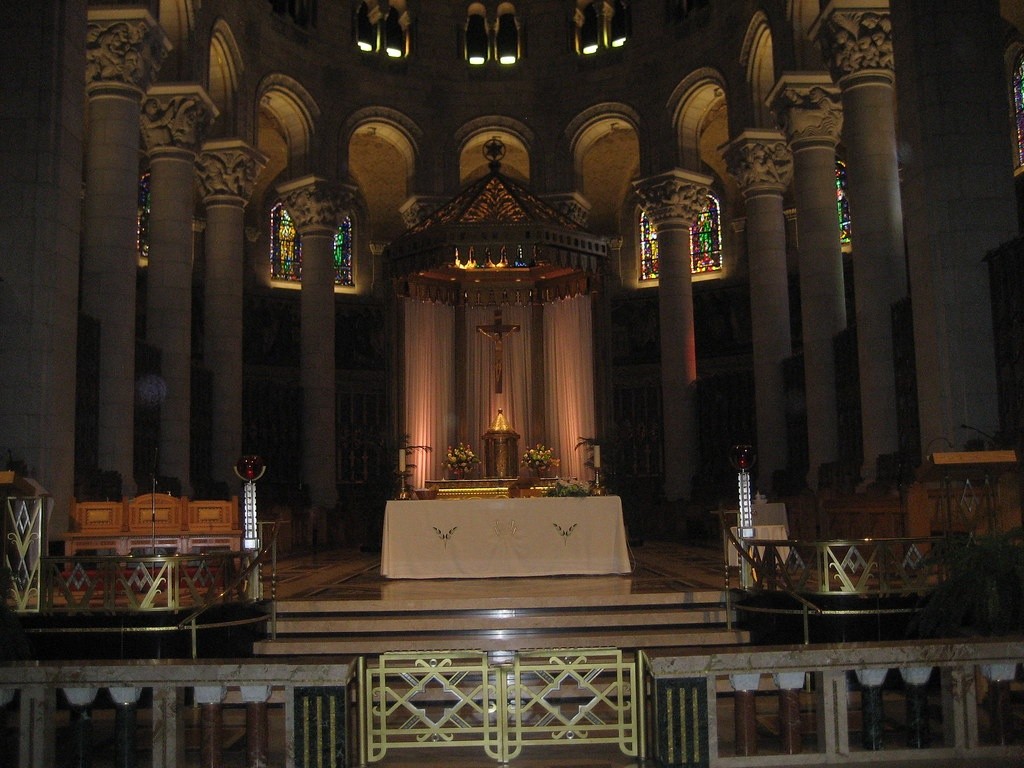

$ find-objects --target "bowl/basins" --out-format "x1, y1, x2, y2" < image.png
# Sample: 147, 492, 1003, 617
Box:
415, 490, 438, 500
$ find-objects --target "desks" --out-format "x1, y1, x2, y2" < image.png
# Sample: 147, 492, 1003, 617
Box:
380, 493, 633, 580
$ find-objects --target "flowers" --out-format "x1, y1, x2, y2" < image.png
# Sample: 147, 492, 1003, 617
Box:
521, 444, 561, 472
447, 442, 480, 472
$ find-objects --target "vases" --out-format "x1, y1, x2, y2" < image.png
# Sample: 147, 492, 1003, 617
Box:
529, 465, 557, 477
454, 467, 477, 479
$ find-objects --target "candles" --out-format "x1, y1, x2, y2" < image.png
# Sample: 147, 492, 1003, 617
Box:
594, 444, 601, 468
